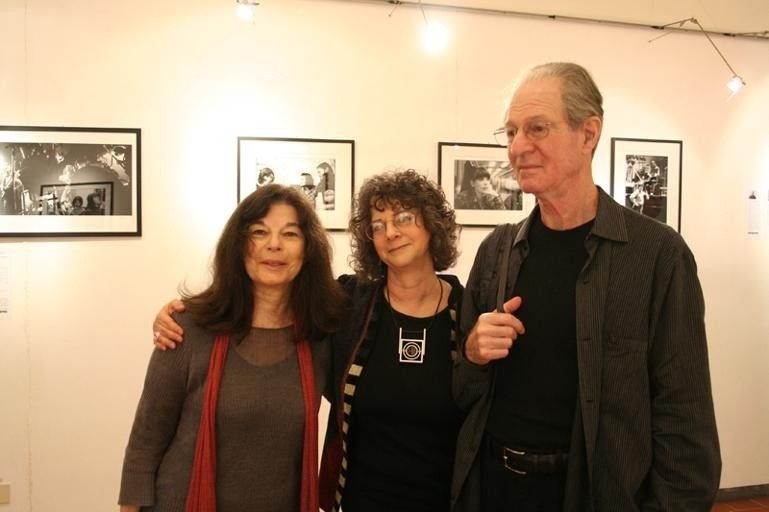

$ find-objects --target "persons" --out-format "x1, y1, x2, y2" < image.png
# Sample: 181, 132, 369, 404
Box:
627, 157, 661, 215
315, 161, 336, 210
80, 192, 107, 215
256, 167, 274, 190
448, 60, 723, 511
150, 166, 467, 510
67, 196, 87, 215
117, 182, 355, 511
454, 161, 505, 210
298, 172, 319, 211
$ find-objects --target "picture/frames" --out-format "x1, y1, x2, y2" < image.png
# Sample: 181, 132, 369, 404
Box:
438, 141, 541, 227
0, 126, 140, 237
610, 139, 683, 235
236, 136, 355, 233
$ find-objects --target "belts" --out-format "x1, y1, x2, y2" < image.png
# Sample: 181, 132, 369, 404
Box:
496, 447, 564, 476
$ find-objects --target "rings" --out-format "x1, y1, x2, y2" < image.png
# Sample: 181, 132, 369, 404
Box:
152, 330, 160, 345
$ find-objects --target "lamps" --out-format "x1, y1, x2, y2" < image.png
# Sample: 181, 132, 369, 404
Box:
653, 13, 746, 99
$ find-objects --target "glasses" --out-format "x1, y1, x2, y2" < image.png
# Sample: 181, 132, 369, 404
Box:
366, 210, 418, 241
493, 121, 563, 146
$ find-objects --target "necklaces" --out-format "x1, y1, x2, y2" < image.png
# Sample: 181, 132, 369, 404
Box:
386, 275, 444, 365
